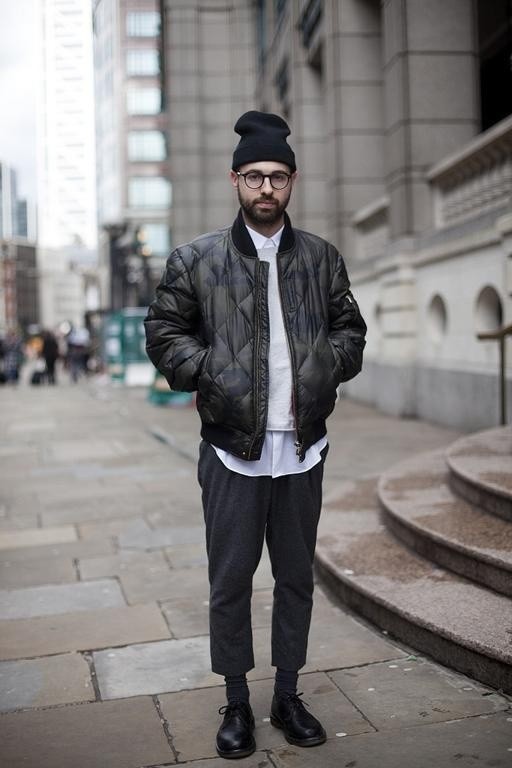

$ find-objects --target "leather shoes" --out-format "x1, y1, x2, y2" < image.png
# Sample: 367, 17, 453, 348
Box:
215, 701, 257, 760
269, 694, 327, 747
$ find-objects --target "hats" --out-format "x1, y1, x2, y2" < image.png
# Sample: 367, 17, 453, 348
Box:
230, 111, 297, 172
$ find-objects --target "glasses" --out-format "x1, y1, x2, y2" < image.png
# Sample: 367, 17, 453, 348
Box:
236, 169, 292, 190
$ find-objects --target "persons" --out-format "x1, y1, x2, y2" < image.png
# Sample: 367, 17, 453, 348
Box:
144, 110, 370, 759
0, 321, 97, 388
